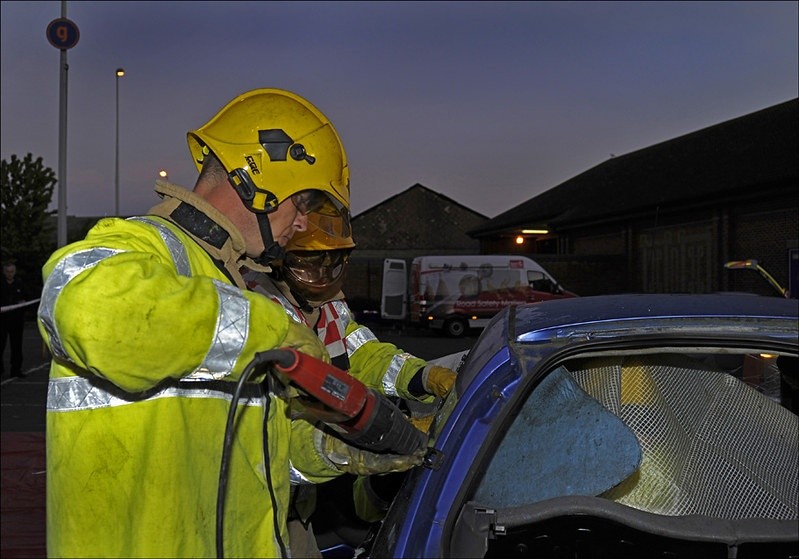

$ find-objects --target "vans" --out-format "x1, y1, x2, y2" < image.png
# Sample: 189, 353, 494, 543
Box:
380, 254, 580, 337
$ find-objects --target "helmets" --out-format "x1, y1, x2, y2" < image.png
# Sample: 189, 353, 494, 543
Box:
184, 87, 351, 212
280, 200, 355, 302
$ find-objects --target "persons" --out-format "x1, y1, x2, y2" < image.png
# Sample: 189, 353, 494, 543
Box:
0, 262, 31, 379
38, 89, 459, 559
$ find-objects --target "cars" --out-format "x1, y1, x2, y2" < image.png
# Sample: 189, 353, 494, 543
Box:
288, 265, 799, 559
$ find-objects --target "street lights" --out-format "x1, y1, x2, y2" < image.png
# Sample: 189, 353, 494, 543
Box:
114, 67, 124, 222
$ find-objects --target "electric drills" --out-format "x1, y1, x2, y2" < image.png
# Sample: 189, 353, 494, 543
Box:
256, 347, 430, 462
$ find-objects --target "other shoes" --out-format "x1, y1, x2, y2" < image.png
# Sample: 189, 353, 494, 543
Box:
9, 368, 28, 379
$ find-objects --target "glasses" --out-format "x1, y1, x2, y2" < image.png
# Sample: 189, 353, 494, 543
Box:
290, 189, 323, 216
289, 259, 344, 284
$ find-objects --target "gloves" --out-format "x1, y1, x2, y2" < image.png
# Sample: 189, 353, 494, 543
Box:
408, 414, 437, 433
421, 363, 458, 399
272, 315, 332, 397
324, 433, 429, 476
